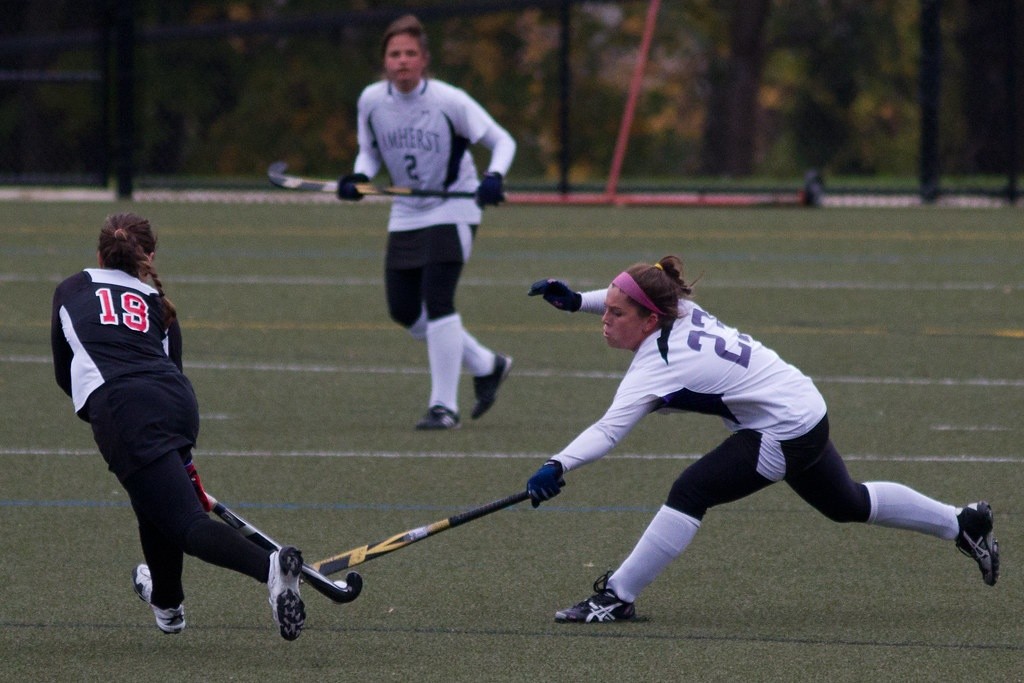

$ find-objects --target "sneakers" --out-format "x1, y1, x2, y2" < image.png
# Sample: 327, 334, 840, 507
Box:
268, 548, 307, 641
471, 354, 512, 419
954, 502, 999, 586
416, 405, 462, 430
554, 570, 636, 623
132, 564, 185, 634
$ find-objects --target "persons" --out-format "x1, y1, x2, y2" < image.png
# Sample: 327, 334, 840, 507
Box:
51, 213, 308, 640
339, 16, 517, 429
525, 254, 998, 624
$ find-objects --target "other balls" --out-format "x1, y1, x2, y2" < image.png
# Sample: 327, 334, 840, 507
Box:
333, 579, 347, 588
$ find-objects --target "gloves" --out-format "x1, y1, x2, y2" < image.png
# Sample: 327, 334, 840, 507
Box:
476, 172, 506, 211
528, 279, 582, 313
526, 459, 565, 509
336, 173, 370, 201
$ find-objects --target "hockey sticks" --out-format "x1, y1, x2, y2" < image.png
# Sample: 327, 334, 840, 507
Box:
204, 491, 363, 603
265, 158, 504, 200
304, 477, 566, 584
